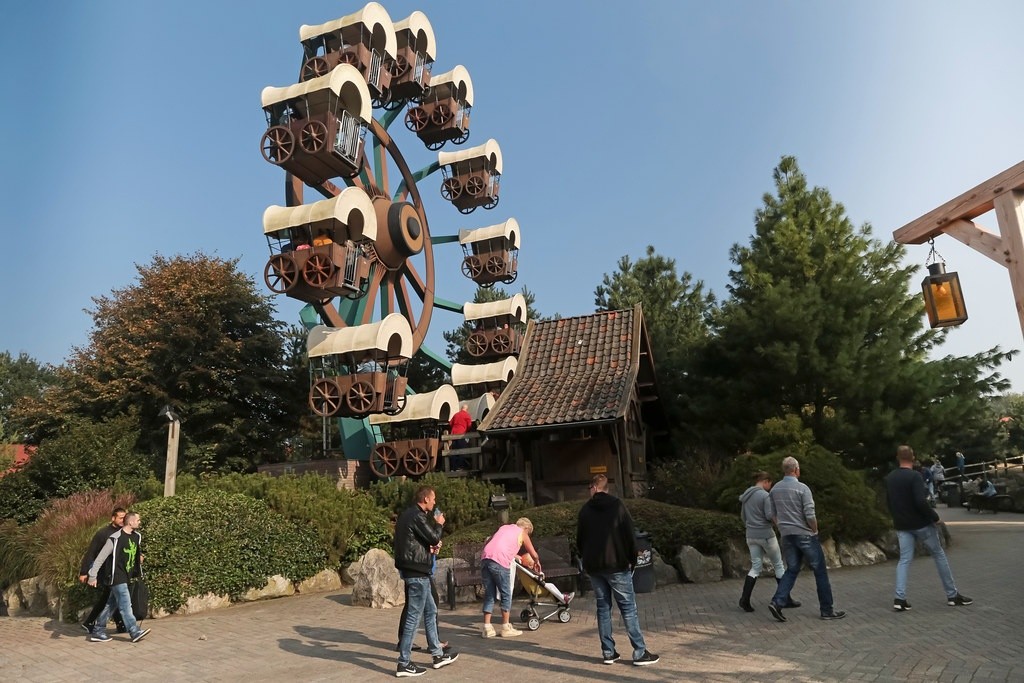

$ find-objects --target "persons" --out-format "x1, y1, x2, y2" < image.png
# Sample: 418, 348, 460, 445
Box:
88, 512, 151, 642
739, 469, 802, 612
930, 459, 946, 500
357, 351, 382, 372
576, 475, 660, 665
449, 403, 472, 472
290, 113, 300, 123
884, 445, 973, 610
521, 552, 575, 605
480, 517, 540, 638
768, 457, 846, 621
78, 508, 125, 633
393, 486, 459, 676
913, 459, 936, 507
490, 388, 500, 401
281, 228, 332, 253
963, 474, 997, 514
475, 322, 508, 331
956, 452, 969, 482
396, 540, 452, 653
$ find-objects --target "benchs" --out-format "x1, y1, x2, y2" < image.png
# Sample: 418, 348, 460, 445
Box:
446, 537, 584, 609
967, 485, 1016, 513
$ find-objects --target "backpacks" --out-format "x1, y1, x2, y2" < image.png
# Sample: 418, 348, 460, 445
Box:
128, 580, 148, 620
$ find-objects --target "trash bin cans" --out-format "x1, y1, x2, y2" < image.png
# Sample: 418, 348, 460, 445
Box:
631, 532, 656, 594
947, 484, 961, 508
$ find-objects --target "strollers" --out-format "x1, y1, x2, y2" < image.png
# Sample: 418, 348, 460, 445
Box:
513, 558, 573, 631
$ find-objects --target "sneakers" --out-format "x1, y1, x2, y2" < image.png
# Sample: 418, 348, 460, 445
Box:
947, 592, 973, 605
91, 632, 113, 642
432, 652, 460, 669
482, 627, 499, 638
603, 652, 621, 665
822, 610, 847, 620
769, 602, 787, 622
894, 599, 912, 611
133, 629, 151, 643
396, 662, 427, 678
501, 626, 523, 637
633, 650, 659, 666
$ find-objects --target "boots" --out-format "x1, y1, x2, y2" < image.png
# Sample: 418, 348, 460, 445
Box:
776, 576, 801, 608
738, 575, 759, 612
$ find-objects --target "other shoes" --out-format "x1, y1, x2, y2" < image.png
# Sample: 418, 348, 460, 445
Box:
567, 592, 574, 604
117, 625, 128, 633
397, 641, 421, 651
963, 502, 971, 506
427, 644, 451, 652
82, 621, 94, 634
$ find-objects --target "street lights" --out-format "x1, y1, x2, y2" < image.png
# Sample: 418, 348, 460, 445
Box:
890, 159, 1024, 349
